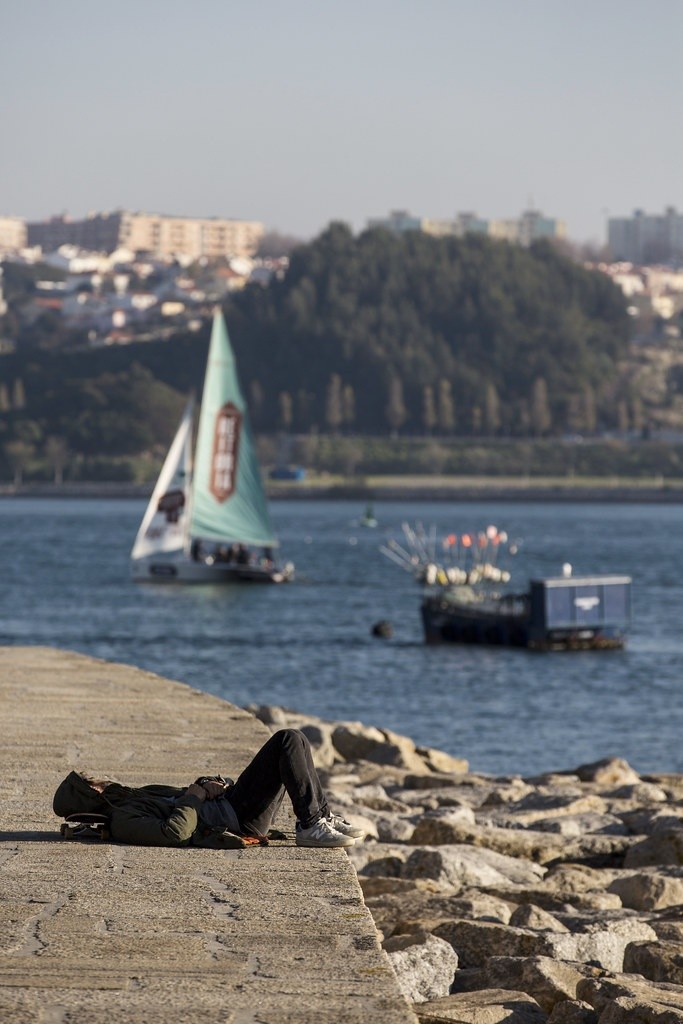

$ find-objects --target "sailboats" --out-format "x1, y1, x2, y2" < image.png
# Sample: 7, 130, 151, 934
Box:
129, 308, 295, 583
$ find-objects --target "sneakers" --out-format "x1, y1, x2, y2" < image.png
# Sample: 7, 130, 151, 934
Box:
295, 811, 364, 847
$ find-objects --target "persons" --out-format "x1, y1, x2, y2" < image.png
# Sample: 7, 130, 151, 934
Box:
53, 727, 365, 850
191, 537, 249, 565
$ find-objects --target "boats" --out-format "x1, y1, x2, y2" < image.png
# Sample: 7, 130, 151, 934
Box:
418, 571, 633, 654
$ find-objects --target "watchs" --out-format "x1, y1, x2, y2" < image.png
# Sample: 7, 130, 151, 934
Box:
201, 779, 210, 787
